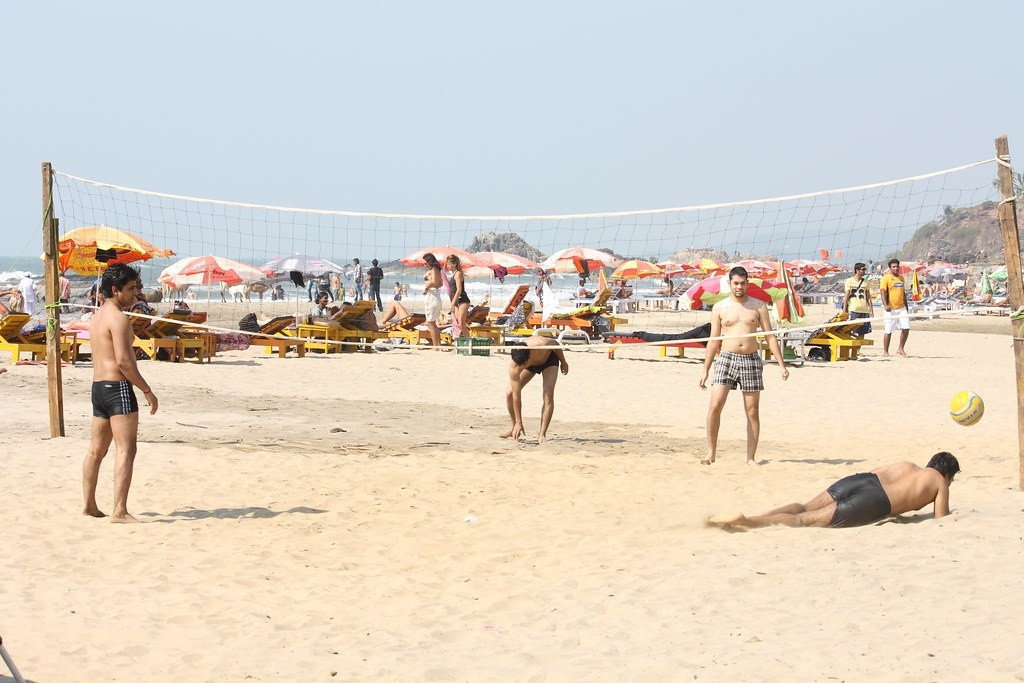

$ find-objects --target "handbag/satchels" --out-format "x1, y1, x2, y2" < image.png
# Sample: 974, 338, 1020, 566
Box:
842, 301, 849, 319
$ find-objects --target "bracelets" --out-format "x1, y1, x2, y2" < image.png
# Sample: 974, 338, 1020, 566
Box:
143, 388, 151, 394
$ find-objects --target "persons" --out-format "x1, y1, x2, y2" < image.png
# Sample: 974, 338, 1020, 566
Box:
802, 277, 810, 287
82, 262, 158, 523
186, 289, 196, 300
573, 279, 633, 298
271, 286, 286, 301
843, 263, 874, 356
880, 259, 911, 357
307, 253, 470, 353
59, 273, 70, 312
0, 271, 37, 318
910, 272, 1011, 307
698, 266, 789, 469
811, 275, 821, 284
655, 277, 673, 294
498, 336, 568, 443
82, 280, 157, 360
710, 451, 959, 530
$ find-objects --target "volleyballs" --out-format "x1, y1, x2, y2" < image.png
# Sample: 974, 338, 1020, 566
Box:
949, 390, 985, 427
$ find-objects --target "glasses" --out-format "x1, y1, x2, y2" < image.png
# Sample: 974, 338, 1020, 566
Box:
424, 262, 428, 265
860, 268, 866, 271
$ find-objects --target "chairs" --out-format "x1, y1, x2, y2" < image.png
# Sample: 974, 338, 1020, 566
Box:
1, 288, 874, 364
908, 291, 1011, 319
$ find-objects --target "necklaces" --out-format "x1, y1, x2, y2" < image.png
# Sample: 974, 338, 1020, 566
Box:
109, 299, 123, 312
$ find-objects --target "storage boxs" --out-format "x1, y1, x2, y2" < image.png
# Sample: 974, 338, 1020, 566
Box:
456, 336, 491, 355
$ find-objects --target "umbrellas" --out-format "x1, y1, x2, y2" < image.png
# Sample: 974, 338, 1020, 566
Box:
263, 252, 345, 328
612, 258, 839, 299
40, 225, 269, 310
542, 247, 621, 308
883, 261, 968, 311
401, 247, 538, 328
988, 264, 1024, 299
